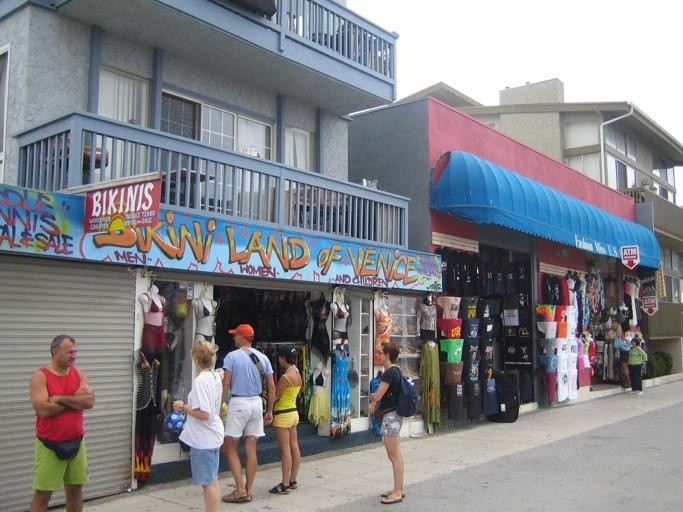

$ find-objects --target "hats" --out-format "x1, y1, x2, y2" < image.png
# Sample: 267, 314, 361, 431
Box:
271, 344, 297, 357
228, 323, 255, 338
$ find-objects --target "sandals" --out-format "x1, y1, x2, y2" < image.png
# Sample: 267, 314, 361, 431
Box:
269, 482, 291, 494
289, 480, 297, 489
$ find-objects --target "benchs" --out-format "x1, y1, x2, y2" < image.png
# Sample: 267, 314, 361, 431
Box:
41, 146, 110, 187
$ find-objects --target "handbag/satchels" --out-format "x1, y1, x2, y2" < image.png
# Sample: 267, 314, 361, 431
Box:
375, 407, 394, 422
601, 306, 631, 360
39, 436, 84, 460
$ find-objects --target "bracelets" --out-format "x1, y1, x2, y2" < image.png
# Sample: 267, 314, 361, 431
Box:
180, 404, 184, 413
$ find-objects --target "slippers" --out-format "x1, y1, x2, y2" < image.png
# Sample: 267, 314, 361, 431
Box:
381, 497, 403, 504
381, 490, 405, 498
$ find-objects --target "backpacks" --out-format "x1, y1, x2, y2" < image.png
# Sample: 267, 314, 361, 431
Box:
390, 366, 417, 417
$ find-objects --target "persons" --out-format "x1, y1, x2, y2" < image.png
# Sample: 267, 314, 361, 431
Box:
191, 289, 217, 344
171, 341, 225, 511
367, 341, 406, 505
627, 338, 648, 395
29, 334, 94, 511
134, 352, 160, 482
267, 346, 304, 494
603, 273, 615, 298
415, 294, 436, 340
329, 297, 350, 345
307, 361, 329, 427
331, 345, 351, 435
138, 284, 165, 349
156, 313, 178, 409
373, 303, 392, 365
566, 270, 596, 386
221, 324, 277, 502
308, 294, 330, 354
420, 339, 440, 433
622, 277, 643, 326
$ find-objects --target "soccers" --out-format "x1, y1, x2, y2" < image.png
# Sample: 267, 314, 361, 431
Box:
164, 413, 185, 432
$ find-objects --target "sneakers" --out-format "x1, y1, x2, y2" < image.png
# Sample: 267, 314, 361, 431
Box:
223, 489, 253, 502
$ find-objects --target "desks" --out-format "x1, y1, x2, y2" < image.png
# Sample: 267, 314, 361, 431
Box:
162, 168, 215, 206
